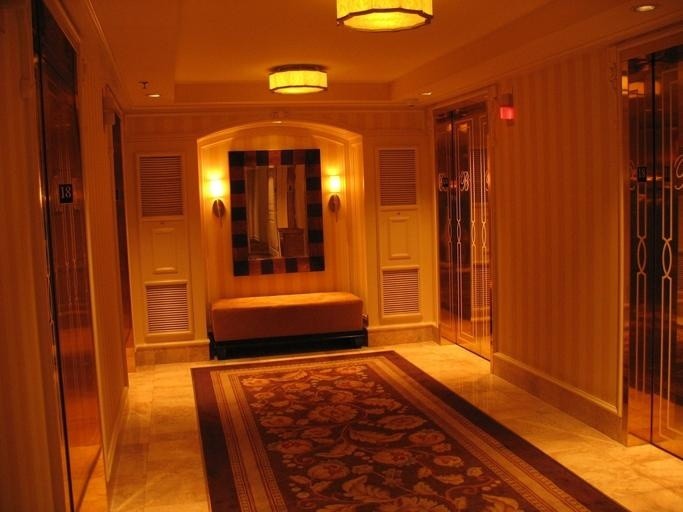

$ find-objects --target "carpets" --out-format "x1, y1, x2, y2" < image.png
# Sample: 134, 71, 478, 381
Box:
188, 349, 629, 512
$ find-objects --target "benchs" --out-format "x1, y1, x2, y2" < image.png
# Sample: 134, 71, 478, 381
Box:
211, 292, 364, 357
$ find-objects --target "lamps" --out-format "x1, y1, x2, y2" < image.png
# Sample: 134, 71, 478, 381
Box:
334, 0, 433, 33
267, 68, 328, 96
498, 93, 511, 119
